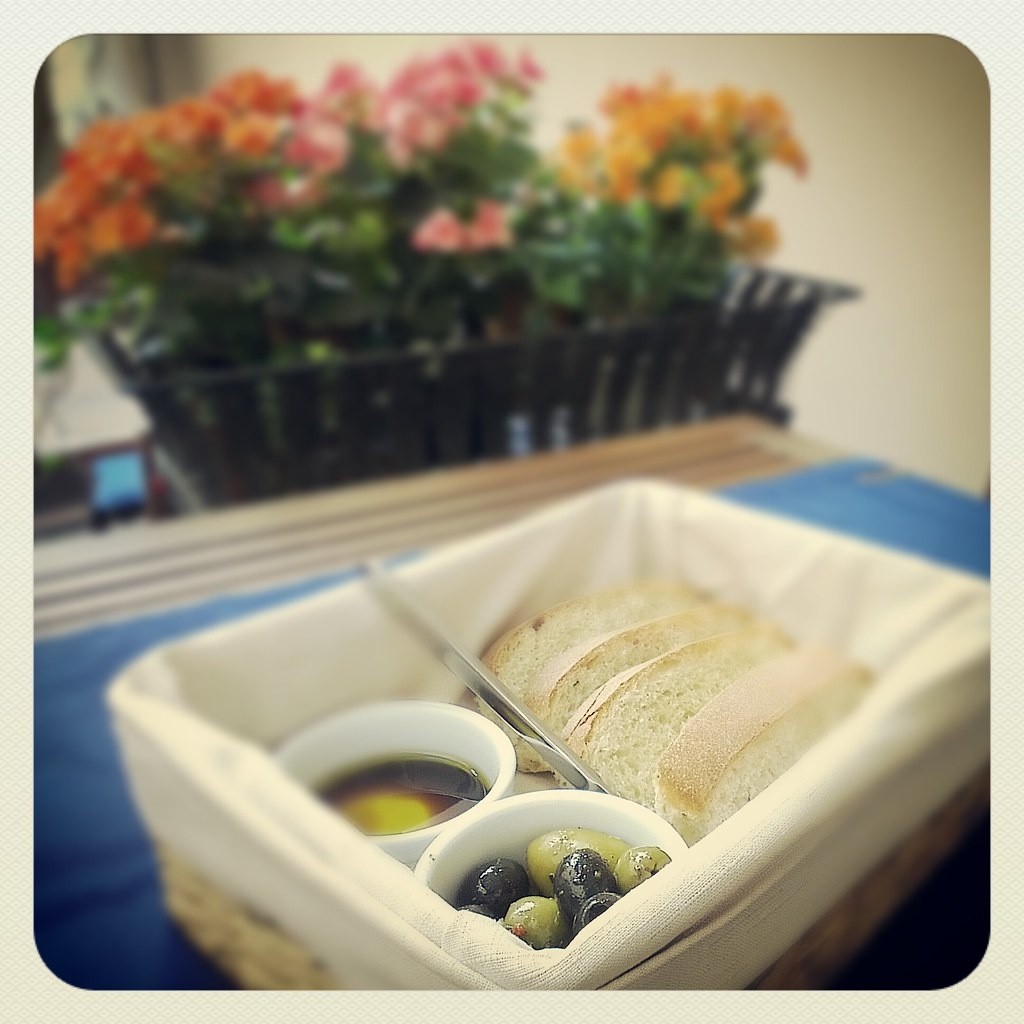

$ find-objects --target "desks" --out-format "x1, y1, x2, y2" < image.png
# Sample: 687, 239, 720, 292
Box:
34, 411, 993, 993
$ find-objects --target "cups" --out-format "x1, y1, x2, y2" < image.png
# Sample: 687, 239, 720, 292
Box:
278, 701, 516, 869
413, 789, 692, 909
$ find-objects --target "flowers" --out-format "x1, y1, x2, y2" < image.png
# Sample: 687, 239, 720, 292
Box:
34, 34, 809, 441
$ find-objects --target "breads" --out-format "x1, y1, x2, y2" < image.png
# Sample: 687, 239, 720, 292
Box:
473, 575, 881, 848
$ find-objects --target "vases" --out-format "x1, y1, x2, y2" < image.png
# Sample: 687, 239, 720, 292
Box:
96, 263, 868, 504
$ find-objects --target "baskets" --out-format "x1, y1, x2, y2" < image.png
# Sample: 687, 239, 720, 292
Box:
104, 478, 992, 988
95, 257, 858, 511
